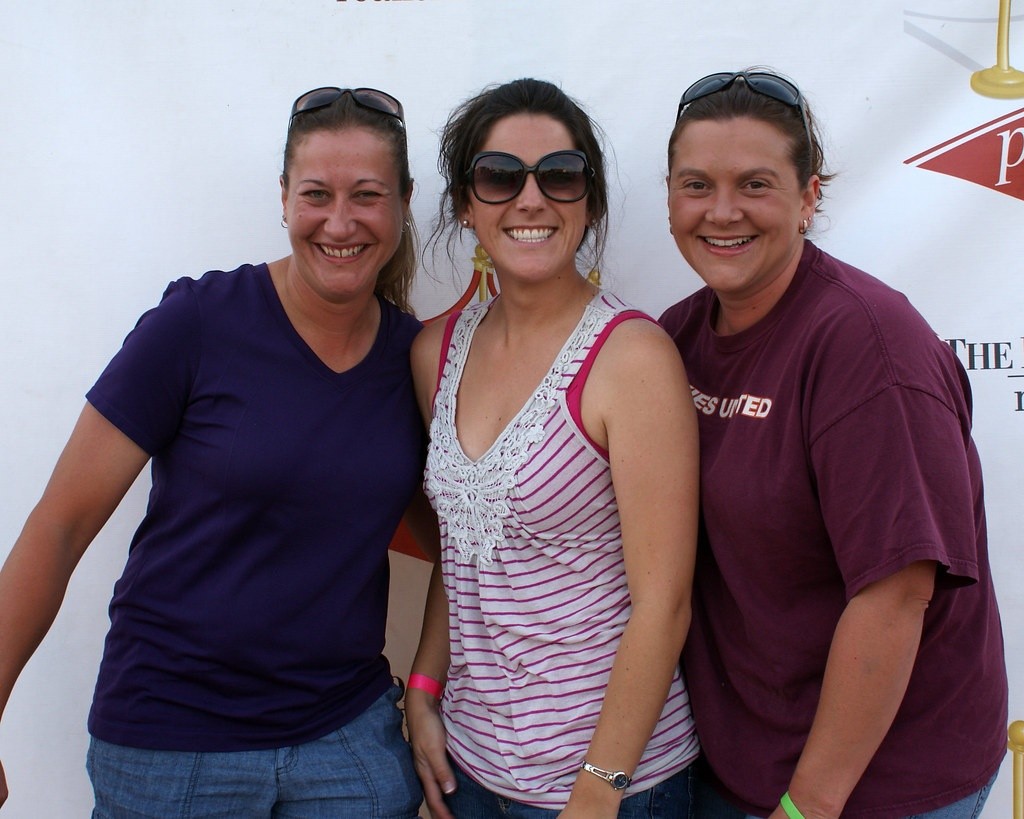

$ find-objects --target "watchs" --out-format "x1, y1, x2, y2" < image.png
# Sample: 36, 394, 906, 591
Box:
581, 761, 632, 790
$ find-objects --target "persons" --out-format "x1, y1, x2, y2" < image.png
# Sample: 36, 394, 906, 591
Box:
404, 76, 703, 819
0, 87, 440, 819
649, 70, 1007, 819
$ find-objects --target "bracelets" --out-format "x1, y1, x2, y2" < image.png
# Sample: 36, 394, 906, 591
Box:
408, 674, 445, 700
780, 791, 805, 819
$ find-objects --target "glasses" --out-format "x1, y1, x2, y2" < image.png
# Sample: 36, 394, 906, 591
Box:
287, 86, 406, 148
676, 71, 813, 151
462, 149, 596, 205
